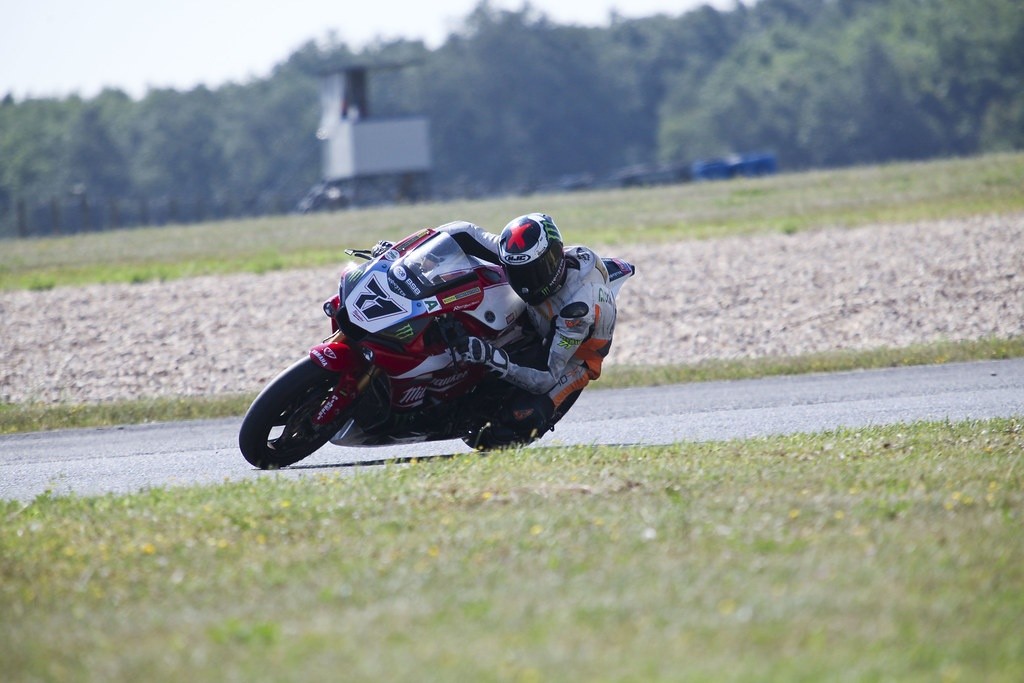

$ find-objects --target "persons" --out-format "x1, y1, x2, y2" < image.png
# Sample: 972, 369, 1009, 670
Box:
371, 213, 615, 450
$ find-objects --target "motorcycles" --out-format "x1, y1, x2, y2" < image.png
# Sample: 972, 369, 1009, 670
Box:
239, 228, 636, 470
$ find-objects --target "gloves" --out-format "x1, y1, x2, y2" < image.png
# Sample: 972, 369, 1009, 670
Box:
448, 335, 510, 379
371, 240, 397, 258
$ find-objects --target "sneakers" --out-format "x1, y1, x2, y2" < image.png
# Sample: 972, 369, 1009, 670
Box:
476, 411, 535, 449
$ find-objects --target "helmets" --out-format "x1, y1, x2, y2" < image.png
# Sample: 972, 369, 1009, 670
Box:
498, 212, 567, 307
